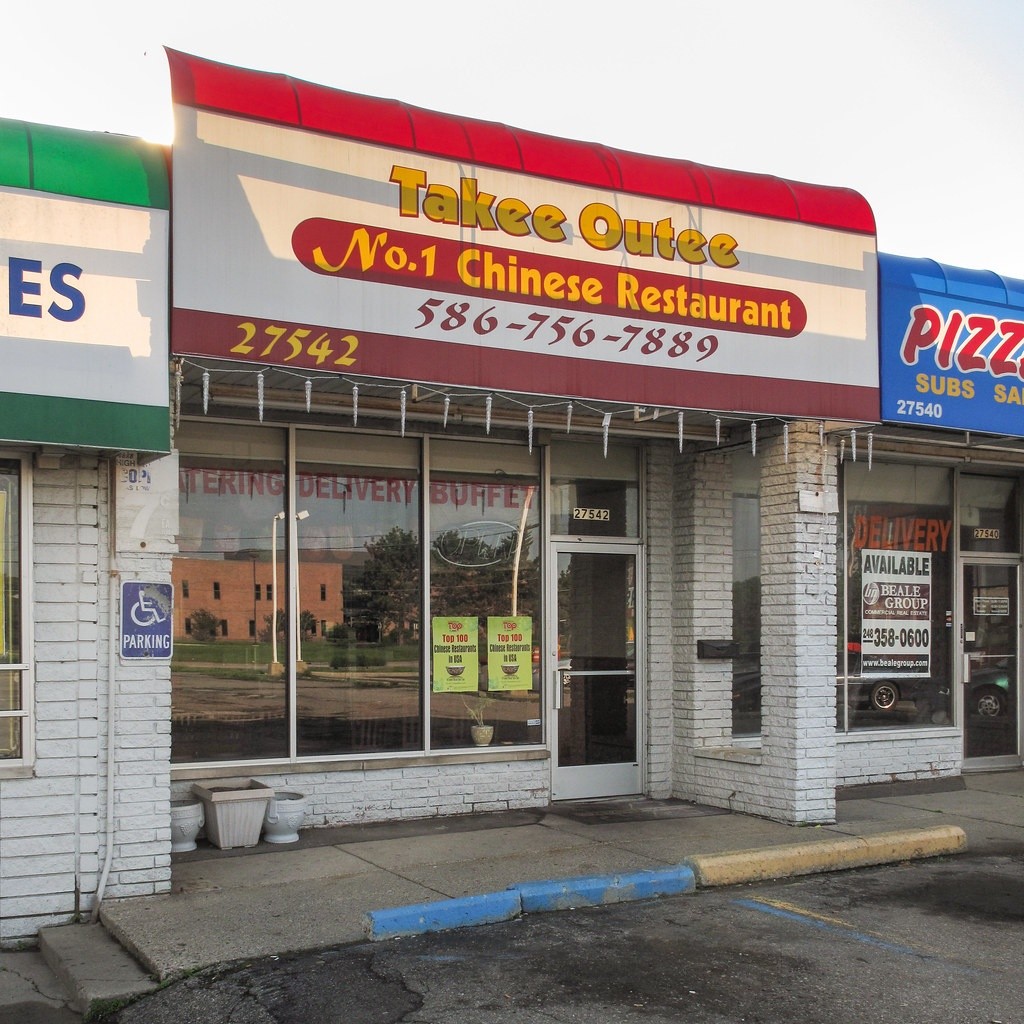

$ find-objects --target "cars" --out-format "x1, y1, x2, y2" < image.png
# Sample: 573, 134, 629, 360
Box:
834, 620, 951, 710
964, 656, 1015, 717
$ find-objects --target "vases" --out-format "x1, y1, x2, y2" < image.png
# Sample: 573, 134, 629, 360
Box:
261, 788, 307, 844
170, 800, 206, 852
190, 779, 275, 852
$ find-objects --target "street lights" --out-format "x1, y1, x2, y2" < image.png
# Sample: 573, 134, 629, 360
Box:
295, 510, 310, 662
271, 511, 286, 663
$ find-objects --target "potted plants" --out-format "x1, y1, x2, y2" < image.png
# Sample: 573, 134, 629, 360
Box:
460, 691, 497, 747
